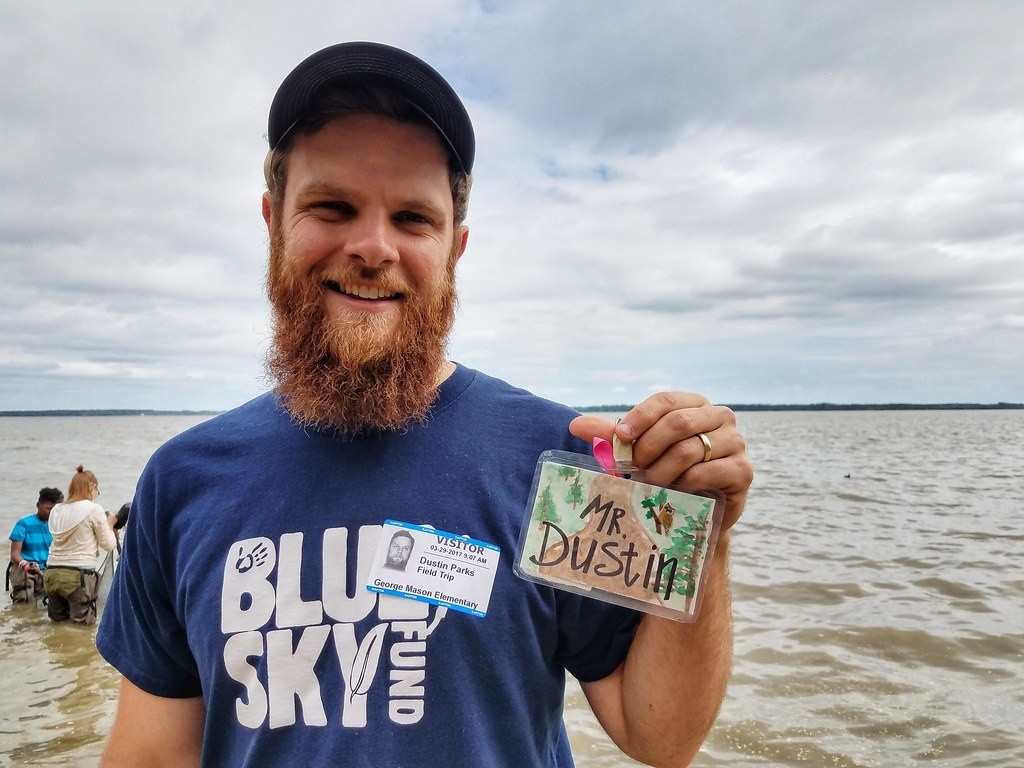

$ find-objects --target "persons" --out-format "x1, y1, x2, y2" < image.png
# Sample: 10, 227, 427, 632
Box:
95, 40, 757, 766
8, 488, 64, 606
43, 465, 119, 626
384, 530, 415, 572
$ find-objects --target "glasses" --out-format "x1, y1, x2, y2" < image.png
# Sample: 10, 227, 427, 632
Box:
90, 484, 100, 496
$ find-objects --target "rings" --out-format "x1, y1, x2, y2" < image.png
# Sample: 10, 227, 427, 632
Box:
697, 433, 713, 463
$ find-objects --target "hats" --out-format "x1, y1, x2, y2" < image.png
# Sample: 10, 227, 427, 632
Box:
269, 40, 475, 173
